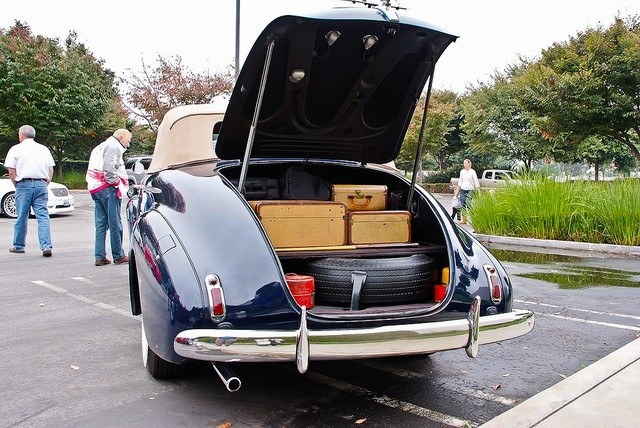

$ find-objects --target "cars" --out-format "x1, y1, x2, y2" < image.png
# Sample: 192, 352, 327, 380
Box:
124, 154, 153, 199
126, 0, 535, 393
0, 162, 74, 219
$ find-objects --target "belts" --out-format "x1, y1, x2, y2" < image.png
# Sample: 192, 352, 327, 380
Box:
23, 178, 46, 181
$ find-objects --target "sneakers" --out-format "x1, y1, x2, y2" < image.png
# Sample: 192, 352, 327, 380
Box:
94, 258, 111, 266
113, 255, 129, 263
42, 249, 52, 257
8, 246, 26, 253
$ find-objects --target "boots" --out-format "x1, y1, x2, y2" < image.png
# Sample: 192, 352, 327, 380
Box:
459, 216, 466, 224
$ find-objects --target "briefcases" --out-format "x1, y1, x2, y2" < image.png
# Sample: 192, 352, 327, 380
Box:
348, 211, 412, 245
331, 184, 388, 212
247, 200, 348, 248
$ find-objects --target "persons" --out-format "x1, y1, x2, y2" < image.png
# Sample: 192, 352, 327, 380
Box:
457, 159, 481, 224
85, 129, 134, 267
4, 125, 57, 256
450, 185, 462, 221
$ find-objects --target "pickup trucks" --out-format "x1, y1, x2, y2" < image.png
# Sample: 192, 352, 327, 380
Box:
449, 169, 534, 193
